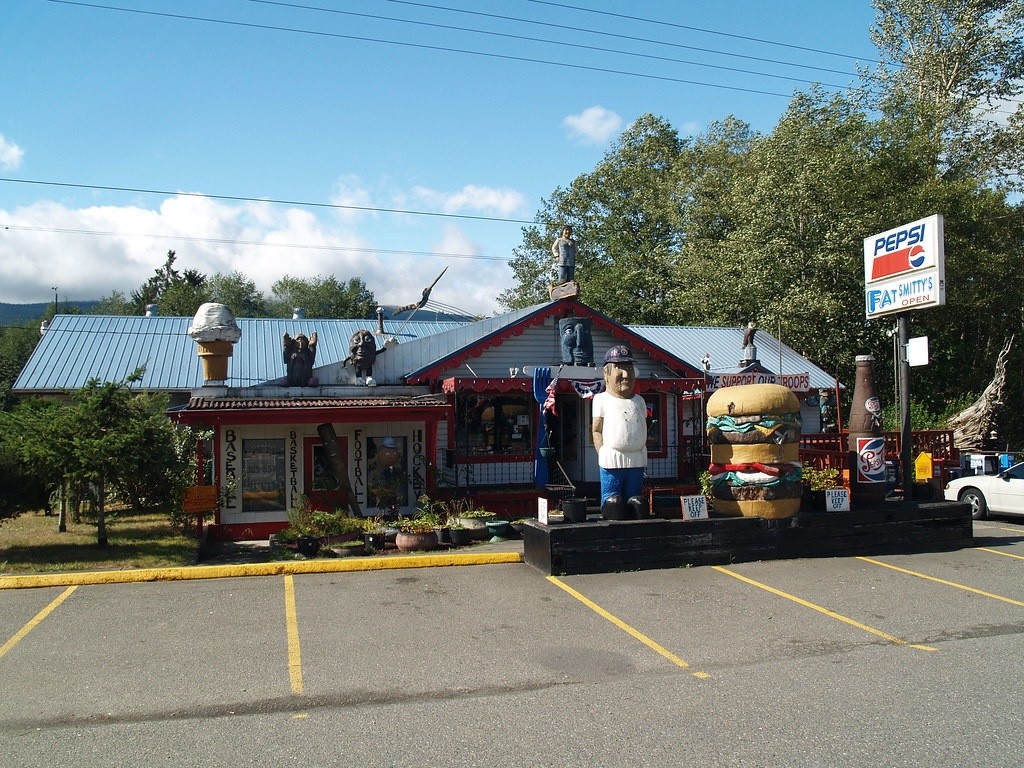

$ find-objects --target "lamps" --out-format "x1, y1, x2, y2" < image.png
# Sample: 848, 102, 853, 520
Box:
510, 367, 519, 378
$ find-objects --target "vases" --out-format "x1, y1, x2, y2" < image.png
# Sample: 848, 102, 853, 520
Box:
539, 447, 554, 457
486, 521, 509, 542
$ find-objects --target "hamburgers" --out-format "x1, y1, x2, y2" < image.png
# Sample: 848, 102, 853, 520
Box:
705, 383, 802, 518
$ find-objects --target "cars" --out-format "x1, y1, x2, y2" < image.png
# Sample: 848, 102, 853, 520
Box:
943, 462, 1024, 521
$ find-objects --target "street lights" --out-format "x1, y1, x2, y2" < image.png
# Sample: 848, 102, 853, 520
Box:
836, 376, 846, 433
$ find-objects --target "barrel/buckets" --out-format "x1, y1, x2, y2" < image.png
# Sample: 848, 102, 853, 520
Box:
562, 499, 587, 522
539, 447, 555, 458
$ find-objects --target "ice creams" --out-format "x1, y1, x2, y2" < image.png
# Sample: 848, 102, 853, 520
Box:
187, 302, 242, 380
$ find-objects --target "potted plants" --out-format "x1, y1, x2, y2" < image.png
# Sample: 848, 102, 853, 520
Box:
280, 492, 525, 557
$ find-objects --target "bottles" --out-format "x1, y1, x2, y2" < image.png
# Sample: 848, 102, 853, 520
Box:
846, 355, 887, 505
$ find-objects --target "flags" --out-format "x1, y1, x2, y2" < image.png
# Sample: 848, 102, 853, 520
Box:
543, 371, 560, 416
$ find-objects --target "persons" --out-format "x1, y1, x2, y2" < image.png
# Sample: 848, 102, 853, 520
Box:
339, 329, 399, 386
592, 346, 648, 520
561, 324, 589, 366
280, 331, 319, 387
552, 225, 578, 285
